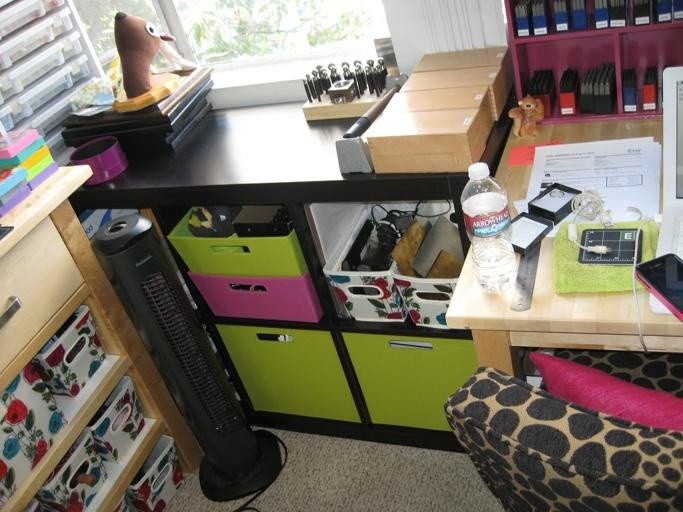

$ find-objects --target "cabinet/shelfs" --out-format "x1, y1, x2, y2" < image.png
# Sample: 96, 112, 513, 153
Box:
152, 182, 517, 453
0, 165, 200, 512
505, 0, 683, 125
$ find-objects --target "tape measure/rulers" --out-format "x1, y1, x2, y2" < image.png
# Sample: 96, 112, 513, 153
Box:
511, 241, 541, 312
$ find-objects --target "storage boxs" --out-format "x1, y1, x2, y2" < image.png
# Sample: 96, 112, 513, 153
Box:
393, 204, 468, 330
0, 305, 186, 512
169, 208, 308, 275
189, 270, 324, 323
325, 203, 402, 324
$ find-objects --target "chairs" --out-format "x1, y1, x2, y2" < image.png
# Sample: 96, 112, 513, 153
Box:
444, 349, 683, 512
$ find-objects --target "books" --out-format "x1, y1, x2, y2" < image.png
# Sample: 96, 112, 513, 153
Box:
61, 65, 214, 152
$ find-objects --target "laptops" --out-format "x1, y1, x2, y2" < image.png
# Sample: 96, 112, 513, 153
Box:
648, 67, 683, 314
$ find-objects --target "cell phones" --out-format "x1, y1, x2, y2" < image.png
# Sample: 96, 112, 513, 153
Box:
634, 254, 683, 322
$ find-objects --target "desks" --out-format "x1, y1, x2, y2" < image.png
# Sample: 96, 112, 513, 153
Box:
446, 121, 683, 376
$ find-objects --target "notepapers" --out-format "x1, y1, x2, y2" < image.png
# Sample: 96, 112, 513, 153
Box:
0, 128, 59, 218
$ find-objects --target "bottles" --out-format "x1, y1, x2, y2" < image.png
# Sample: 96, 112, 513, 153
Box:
458, 161, 517, 298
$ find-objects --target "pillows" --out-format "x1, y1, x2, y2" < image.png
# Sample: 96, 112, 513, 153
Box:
531, 352, 683, 432
